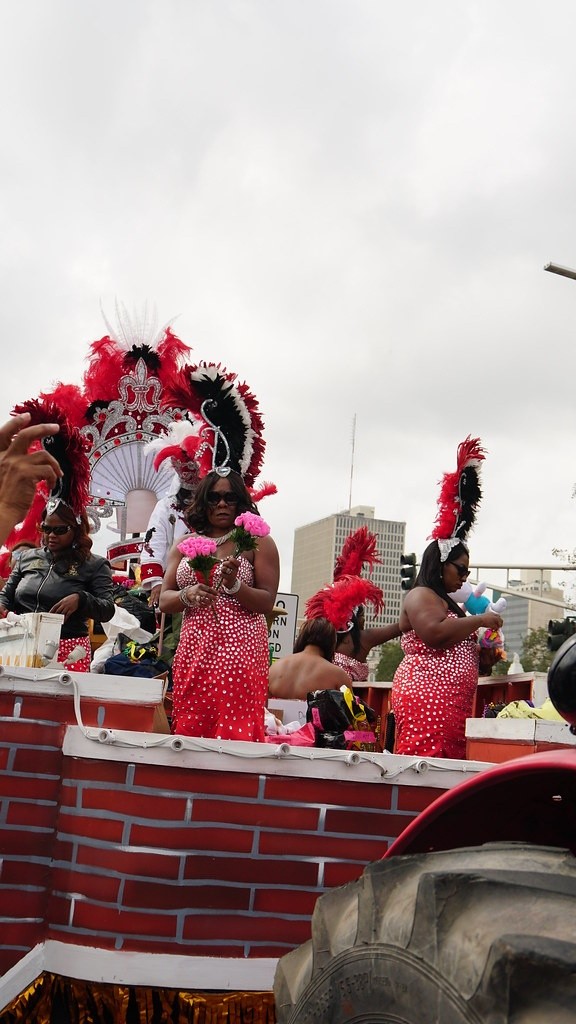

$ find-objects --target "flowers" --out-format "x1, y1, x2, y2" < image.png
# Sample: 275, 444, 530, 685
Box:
218, 511, 270, 591
176, 537, 221, 626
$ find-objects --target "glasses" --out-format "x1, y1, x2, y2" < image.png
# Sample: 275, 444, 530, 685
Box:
206, 491, 239, 506
448, 561, 471, 577
42, 522, 72, 535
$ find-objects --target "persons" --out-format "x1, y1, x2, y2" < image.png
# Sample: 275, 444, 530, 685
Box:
0, 505, 115, 673
391, 537, 503, 759
332, 601, 404, 682
268, 618, 354, 700
0, 543, 36, 592
139, 487, 199, 645
159, 467, 280, 741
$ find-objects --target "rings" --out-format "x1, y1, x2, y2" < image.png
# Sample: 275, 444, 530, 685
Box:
226, 568, 232, 574
196, 596, 200, 601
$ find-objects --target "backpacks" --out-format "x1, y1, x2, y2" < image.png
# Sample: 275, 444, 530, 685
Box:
305, 689, 376, 752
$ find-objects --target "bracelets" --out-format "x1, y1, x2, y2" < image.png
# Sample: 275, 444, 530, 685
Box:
2, 609, 16, 618
222, 577, 241, 594
179, 585, 193, 606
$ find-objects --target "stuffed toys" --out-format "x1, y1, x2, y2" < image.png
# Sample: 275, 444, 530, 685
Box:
447, 579, 509, 614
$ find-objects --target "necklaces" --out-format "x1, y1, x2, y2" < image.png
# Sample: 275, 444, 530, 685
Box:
200, 528, 236, 545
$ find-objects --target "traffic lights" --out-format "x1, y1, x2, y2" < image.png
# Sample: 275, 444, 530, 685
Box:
399, 552, 418, 591
546, 616, 576, 651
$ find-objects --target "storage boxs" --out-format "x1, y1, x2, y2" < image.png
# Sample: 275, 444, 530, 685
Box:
0, 613, 65, 669
